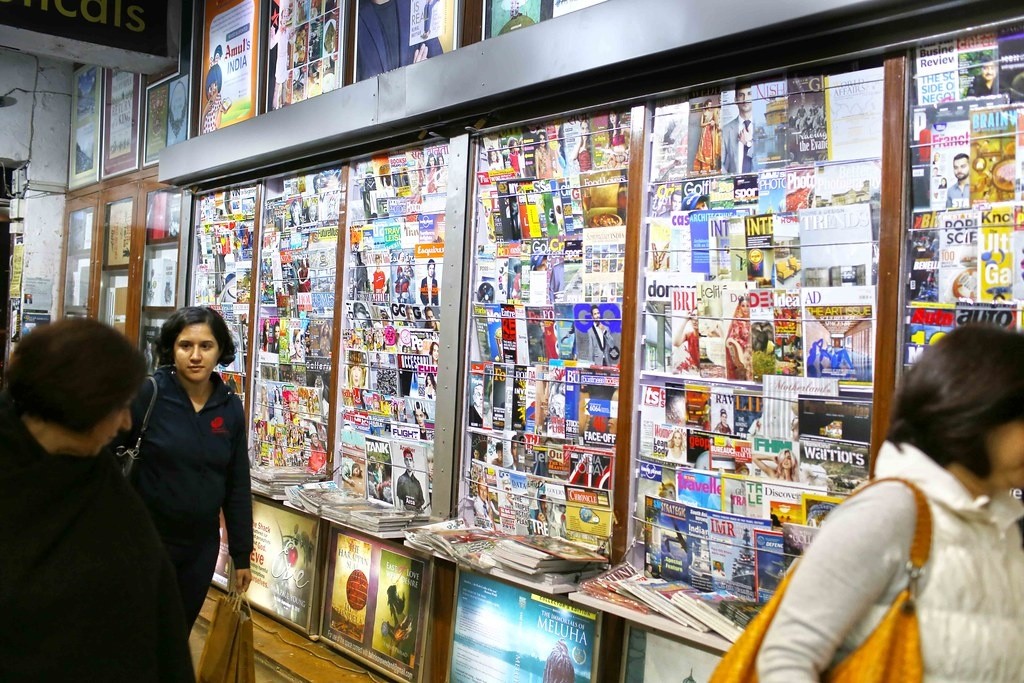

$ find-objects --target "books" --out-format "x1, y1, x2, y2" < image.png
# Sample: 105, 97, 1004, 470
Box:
138, 18, 1024, 646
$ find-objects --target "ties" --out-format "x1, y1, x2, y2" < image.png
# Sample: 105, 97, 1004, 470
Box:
742, 120, 752, 173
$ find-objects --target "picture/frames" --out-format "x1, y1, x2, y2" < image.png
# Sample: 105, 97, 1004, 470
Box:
67, 65, 99, 193
101, 66, 141, 182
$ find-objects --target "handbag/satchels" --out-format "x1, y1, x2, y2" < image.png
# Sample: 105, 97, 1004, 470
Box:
707, 477, 933, 683
195, 581, 255, 683
117, 377, 158, 476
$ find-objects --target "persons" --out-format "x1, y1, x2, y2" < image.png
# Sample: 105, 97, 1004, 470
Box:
0, 316, 196, 683
752, 448, 799, 482
413, 402, 429, 439
507, 435, 525, 472
534, 131, 552, 178
342, 457, 361, 486
420, 259, 438, 305
425, 373, 437, 399
693, 99, 718, 171
396, 449, 425, 511
667, 430, 686, 461
429, 342, 439, 364
424, 307, 440, 331
713, 562, 725, 577
307, 424, 327, 472
492, 442, 503, 467
349, 365, 365, 388
758, 326, 1024, 683
474, 477, 494, 523
111, 306, 252, 638
722, 87, 754, 174
713, 409, 732, 434
403, 305, 417, 328
